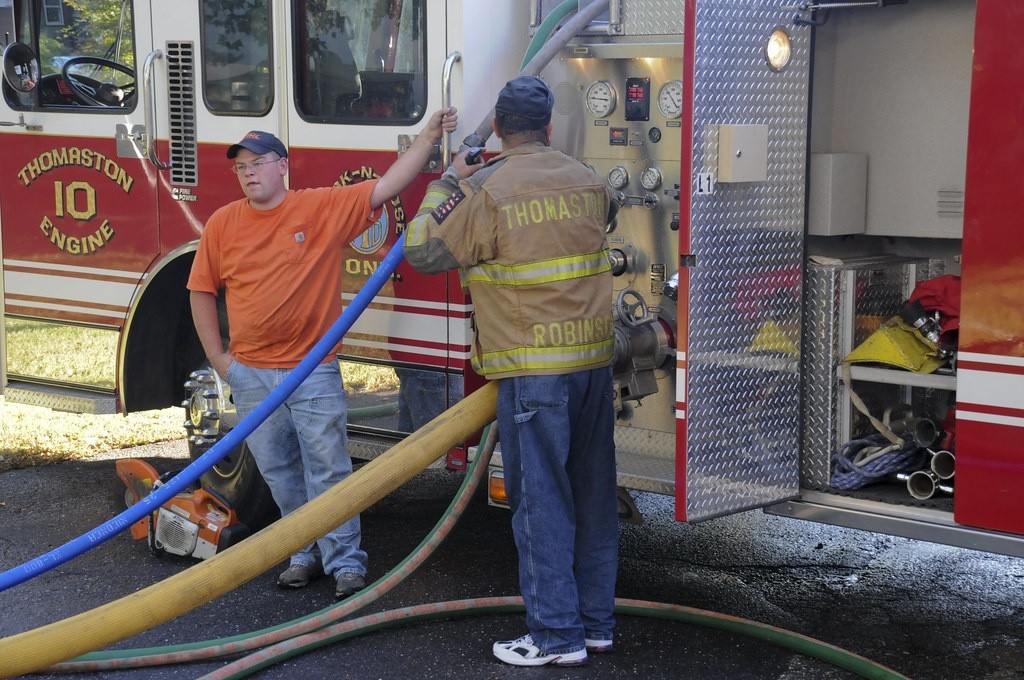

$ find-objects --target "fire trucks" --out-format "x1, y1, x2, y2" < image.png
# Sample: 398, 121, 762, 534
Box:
0, 1, 1023, 560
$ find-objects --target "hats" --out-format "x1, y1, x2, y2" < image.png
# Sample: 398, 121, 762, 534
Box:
227, 131, 287, 160
495, 75, 554, 130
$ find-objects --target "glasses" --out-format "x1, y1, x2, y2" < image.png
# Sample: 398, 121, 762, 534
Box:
231, 159, 277, 174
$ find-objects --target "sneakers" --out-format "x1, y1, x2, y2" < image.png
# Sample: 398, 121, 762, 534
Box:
493, 634, 588, 666
336, 572, 365, 597
585, 638, 613, 653
277, 564, 323, 587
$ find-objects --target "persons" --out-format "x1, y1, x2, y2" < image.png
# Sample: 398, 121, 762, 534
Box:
184, 106, 459, 599
310, 10, 362, 119
401, 77, 619, 665
